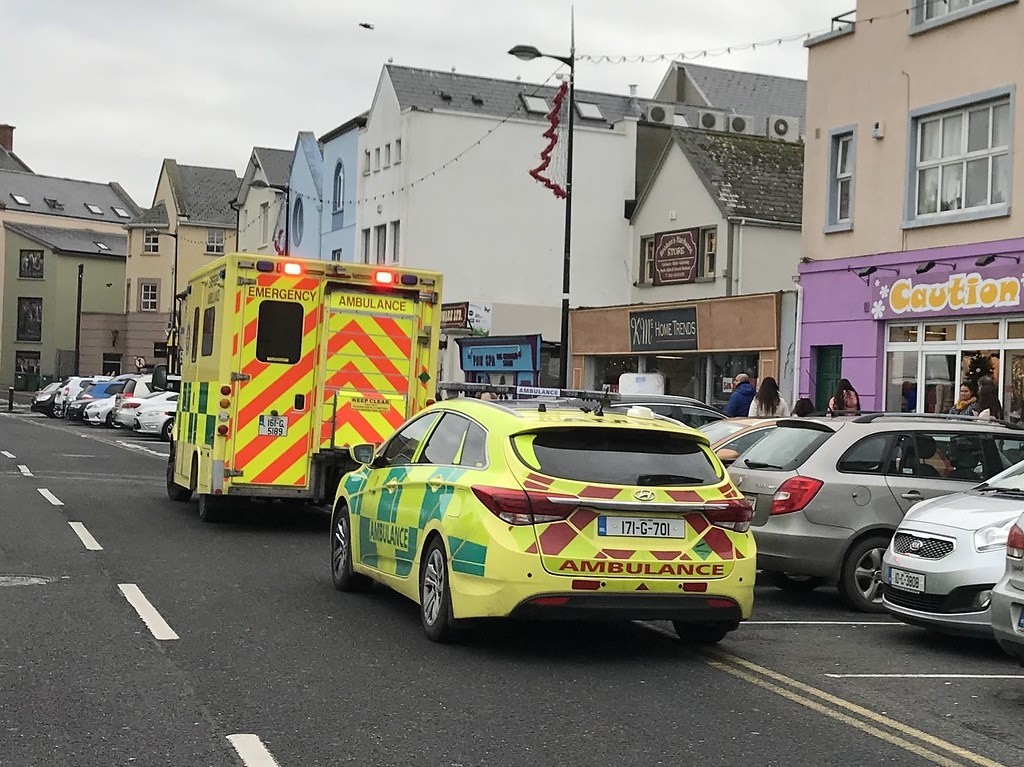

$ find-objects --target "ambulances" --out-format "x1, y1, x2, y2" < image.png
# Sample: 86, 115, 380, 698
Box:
167, 253, 445, 528
329, 379, 759, 649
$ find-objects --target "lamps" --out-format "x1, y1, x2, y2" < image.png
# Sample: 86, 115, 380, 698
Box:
669, 208, 676, 219
110, 327, 120, 347
872, 120, 885, 138
916, 261, 956, 275
859, 266, 900, 277
975, 253, 1020, 267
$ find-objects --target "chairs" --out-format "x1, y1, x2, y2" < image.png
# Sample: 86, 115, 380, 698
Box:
900, 434, 941, 478
946, 440, 983, 480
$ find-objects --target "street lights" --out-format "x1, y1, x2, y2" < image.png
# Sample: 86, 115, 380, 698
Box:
504, 5, 574, 394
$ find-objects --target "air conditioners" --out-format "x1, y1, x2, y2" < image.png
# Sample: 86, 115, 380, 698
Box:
697, 110, 724, 130
646, 103, 675, 125
726, 114, 755, 135
766, 114, 800, 142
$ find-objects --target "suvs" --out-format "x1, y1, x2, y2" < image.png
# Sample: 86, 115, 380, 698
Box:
612, 393, 730, 426
724, 410, 1024, 611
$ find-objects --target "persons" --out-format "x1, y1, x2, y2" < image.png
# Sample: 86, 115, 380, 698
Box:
976, 377, 1003, 420
791, 397, 814, 419
946, 381, 984, 470
826, 378, 861, 417
725, 374, 756, 417
747, 376, 790, 417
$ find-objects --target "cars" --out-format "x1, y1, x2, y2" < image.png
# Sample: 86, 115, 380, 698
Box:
879, 457, 1024, 641
691, 418, 955, 478
29, 373, 181, 443
